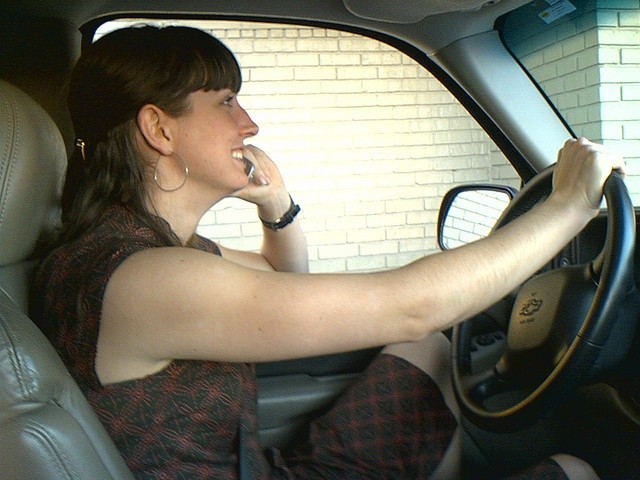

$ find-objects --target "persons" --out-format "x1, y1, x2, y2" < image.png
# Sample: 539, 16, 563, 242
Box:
25, 26, 628, 480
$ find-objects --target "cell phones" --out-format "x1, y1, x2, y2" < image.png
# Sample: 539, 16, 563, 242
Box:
244, 158, 254, 179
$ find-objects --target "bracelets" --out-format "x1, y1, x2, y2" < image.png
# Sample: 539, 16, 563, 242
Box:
258, 196, 300, 233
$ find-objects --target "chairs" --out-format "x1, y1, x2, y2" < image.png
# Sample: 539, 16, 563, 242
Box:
3, 84, 139, 479
